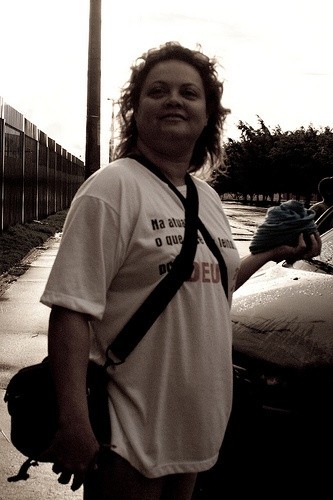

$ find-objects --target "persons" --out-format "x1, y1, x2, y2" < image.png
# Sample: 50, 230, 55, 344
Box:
309, 177, 333, 234
39, 40, 322, 500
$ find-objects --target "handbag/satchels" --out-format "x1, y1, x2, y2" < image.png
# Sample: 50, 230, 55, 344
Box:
5, 351, 111, 482
